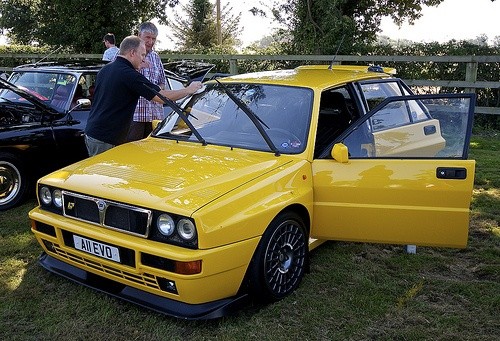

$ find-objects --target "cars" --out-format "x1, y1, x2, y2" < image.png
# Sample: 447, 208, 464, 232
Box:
27, 33, 476, 321
0, 59, 214, 211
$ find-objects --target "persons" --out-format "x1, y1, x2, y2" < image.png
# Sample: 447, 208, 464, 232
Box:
85, 35, 203, 157
102, 33, 120, 60
126, 22, 165, 143
